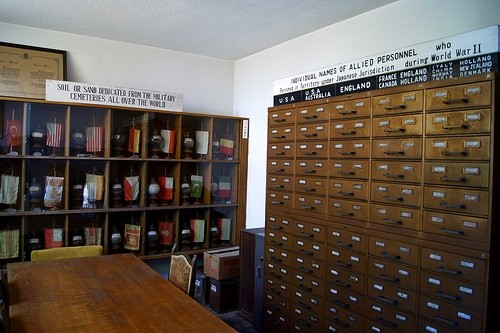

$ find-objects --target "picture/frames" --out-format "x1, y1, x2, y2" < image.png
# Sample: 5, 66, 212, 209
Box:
0, 41, 67, 101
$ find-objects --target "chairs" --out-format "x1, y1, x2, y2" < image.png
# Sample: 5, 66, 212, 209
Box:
168, 255, 197, 295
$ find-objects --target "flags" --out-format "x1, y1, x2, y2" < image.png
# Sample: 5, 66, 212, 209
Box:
6, 119, 22, 145
45, 229, 63, 249
86, 127, 103, 152
43, 176, 64, 207
125, 128, 140, 154
191, 175, 203, 197
46, 122, 63, 147
84, 226, 101, 245
160, 129, 175, 153
219, 133, 234, 156
218, 218, 231, 240
190, 219, 205, 242
158, 221, 174, 244
0, 174, 20, 204
124, 176, 140, 200
158, 176, 173, 199
196, 131, 209, 154
0, 230, 20, 259
85, 174, 104, 200
124, 224, 141, 250
219, 176, 230, 197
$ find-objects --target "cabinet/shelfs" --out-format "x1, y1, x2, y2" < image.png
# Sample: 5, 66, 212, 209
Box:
0, 98, 250, 266
239, 226, 265, 332
262, 71, 500, 333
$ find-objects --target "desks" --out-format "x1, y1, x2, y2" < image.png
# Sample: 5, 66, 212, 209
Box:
8, 254, 238, 333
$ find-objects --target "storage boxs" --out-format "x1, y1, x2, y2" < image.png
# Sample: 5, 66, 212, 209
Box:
203, 246, 239, 280
